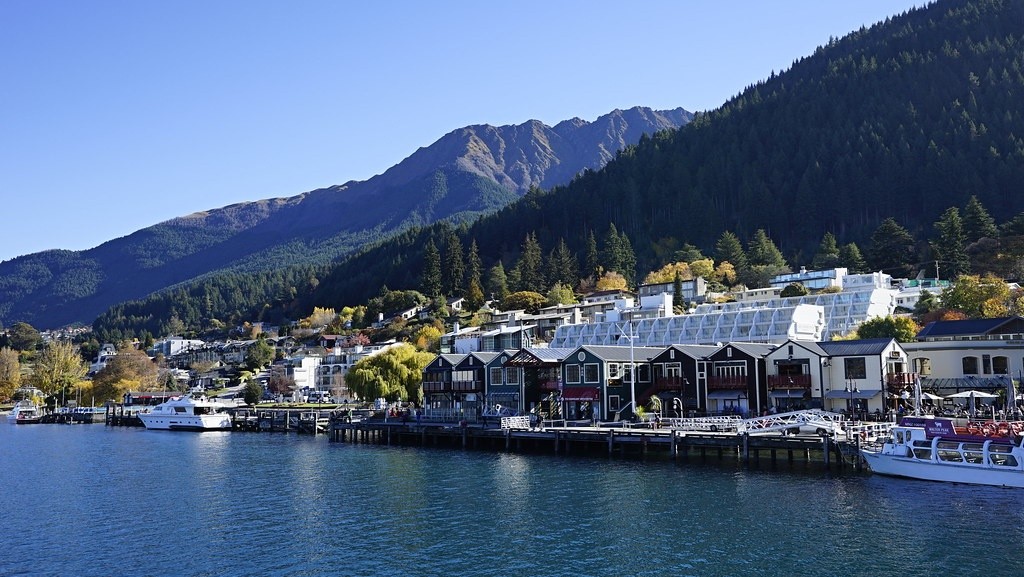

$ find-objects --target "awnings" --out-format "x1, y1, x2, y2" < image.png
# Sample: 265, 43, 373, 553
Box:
707, 389, 741, 399
561, 386, 599, 402
825, 390, 881, 400
768, 389, 806, 398
919, 378, 1009, 390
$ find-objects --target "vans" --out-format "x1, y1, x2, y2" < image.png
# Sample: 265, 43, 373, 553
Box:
306, 390, 330, 403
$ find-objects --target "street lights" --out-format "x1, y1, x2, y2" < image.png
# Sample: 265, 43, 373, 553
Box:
844, 371, 861, 431
673, 397, 684, 418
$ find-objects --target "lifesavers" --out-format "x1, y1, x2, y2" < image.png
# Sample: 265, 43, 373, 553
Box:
982, 422, 997, 437
1011, 422, 1021, 436
966, 422, 981, 436
996, 422, 1011, 437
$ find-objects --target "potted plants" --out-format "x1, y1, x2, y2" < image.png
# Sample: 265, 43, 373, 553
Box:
635, 405, 646, 423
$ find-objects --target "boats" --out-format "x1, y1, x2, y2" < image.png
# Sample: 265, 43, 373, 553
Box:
11, 398, 40, 424
859, 376, 1024, 489
137, 371, 234, 431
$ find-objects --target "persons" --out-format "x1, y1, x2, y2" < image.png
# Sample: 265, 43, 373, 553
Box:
830, 403, 1024, 423
722, 401, 810, 419
388, 407, 421, 426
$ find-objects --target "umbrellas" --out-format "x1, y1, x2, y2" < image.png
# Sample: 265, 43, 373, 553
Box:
888, 389, 1024, 418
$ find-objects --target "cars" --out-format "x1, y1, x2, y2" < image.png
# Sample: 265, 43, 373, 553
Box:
777, 414, 840, 437
703, 416, 737, 432
